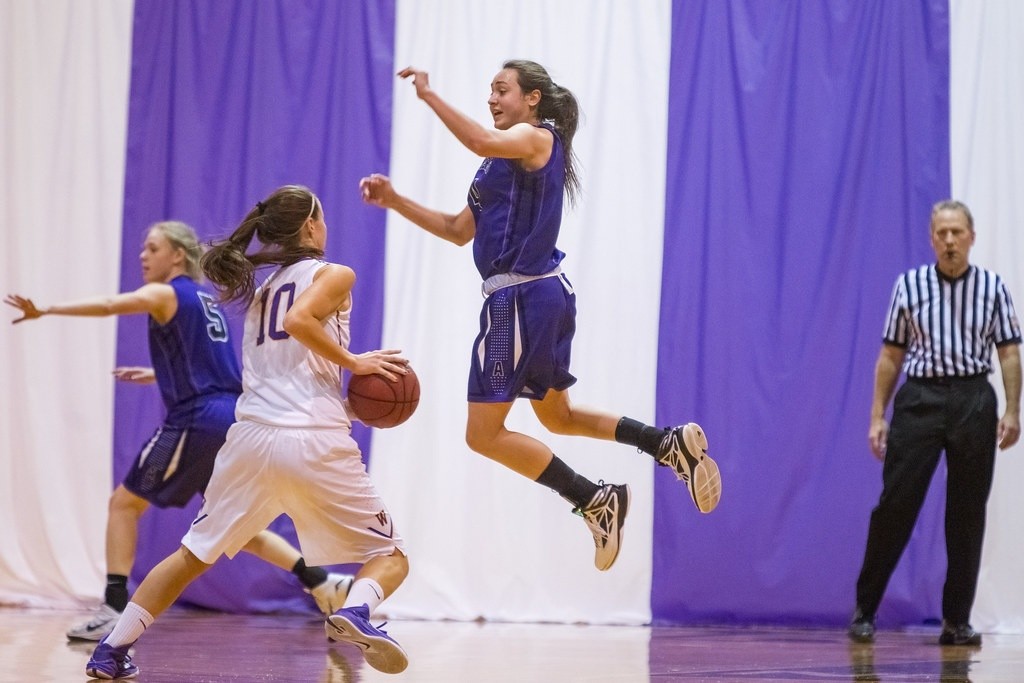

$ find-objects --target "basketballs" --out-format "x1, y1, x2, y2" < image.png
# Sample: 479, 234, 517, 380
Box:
348, 361, 422, 429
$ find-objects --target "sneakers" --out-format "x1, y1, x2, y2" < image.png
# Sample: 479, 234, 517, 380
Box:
324, 602, 408, 674
572, 479, 632, 571
66, 603, 122, 641
86, 625, 140, 680
654, 423, 722, 514
303, 572, 355, 643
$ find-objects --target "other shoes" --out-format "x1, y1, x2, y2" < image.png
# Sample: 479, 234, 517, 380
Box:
939, 624, 983, 646
851, 607, 874, 642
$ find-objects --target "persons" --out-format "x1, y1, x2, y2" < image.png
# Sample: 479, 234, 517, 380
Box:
360, 61, 722, 571
88, 186, 410, 679
2, 222, 355, 645
851, 201, 1022, 645
